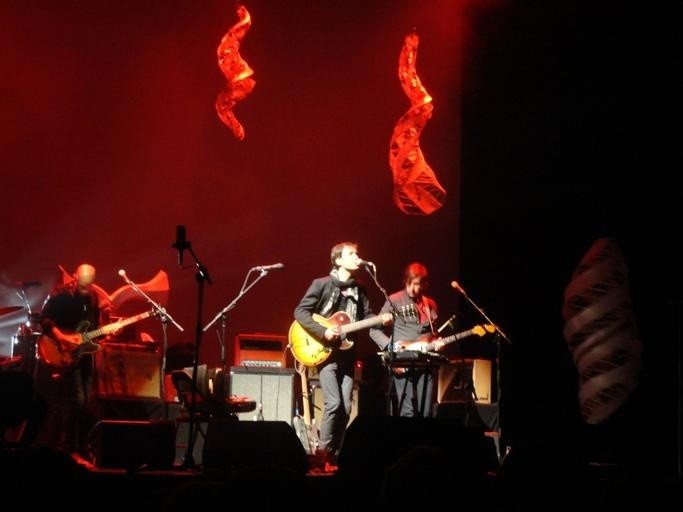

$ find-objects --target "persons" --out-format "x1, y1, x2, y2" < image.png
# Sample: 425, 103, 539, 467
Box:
366, 260, 450, 420
292, 239, 399, 474
37, 263, 123, 428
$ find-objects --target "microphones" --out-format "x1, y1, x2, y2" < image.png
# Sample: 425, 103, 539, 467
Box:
437, 315, 456, 335
256, 262, 284, 272
116, 268, 128, 283
252, 402, 266, 419
354, 257, 375, 268
449, 278, 469, 296
174, 222, 187, 267
14, 280, 43, 289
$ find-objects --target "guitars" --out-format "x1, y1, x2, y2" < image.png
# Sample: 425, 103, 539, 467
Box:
292, 363, 320, 455
287, 304, 418, 367
36, 308, 165, 367
389, 325, 496, 374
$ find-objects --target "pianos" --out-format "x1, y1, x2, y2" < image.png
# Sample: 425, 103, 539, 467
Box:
384, 349, 449, 366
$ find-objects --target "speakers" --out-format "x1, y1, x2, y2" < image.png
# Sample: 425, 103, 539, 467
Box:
227, 366, 297, 425
91, 416, 176, 468
94, 351, 164, 403
